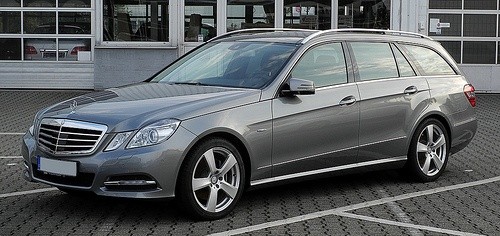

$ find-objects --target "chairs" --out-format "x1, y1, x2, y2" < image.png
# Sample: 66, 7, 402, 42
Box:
71, 46, 90, 61
16, 46, 37, 60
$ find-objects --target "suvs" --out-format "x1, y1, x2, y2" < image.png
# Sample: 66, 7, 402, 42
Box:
21, 28, 478, 221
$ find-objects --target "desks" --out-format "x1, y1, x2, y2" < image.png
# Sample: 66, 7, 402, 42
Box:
40, 49, 68, 59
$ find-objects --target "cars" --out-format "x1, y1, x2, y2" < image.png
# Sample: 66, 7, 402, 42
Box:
25, 25, 90, 62
134, 21, 162, 40
184, 15, 216, 38
